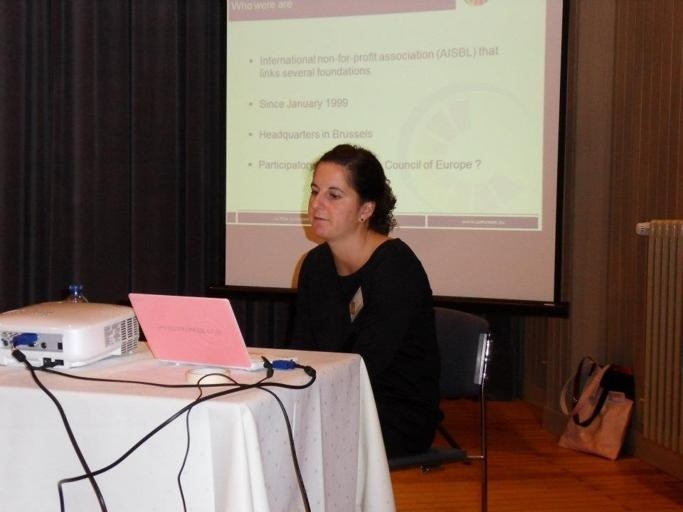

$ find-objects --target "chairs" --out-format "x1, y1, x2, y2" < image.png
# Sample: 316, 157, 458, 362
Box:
381, 307, 495, 512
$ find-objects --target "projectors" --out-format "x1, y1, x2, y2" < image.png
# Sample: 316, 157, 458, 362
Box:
0, 300, 140, 370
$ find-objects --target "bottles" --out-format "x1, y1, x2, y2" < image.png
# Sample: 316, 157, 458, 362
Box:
66, 281, 89, 304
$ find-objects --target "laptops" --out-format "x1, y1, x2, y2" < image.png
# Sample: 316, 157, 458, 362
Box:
128, 293, 297, 372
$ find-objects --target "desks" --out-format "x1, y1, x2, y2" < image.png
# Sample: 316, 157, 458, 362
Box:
1, 340, 365, 511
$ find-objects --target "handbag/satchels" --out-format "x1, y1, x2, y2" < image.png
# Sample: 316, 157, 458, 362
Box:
555, 355, 634, 463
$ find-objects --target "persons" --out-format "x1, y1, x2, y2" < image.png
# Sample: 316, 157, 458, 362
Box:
279, 143, 445, 459
